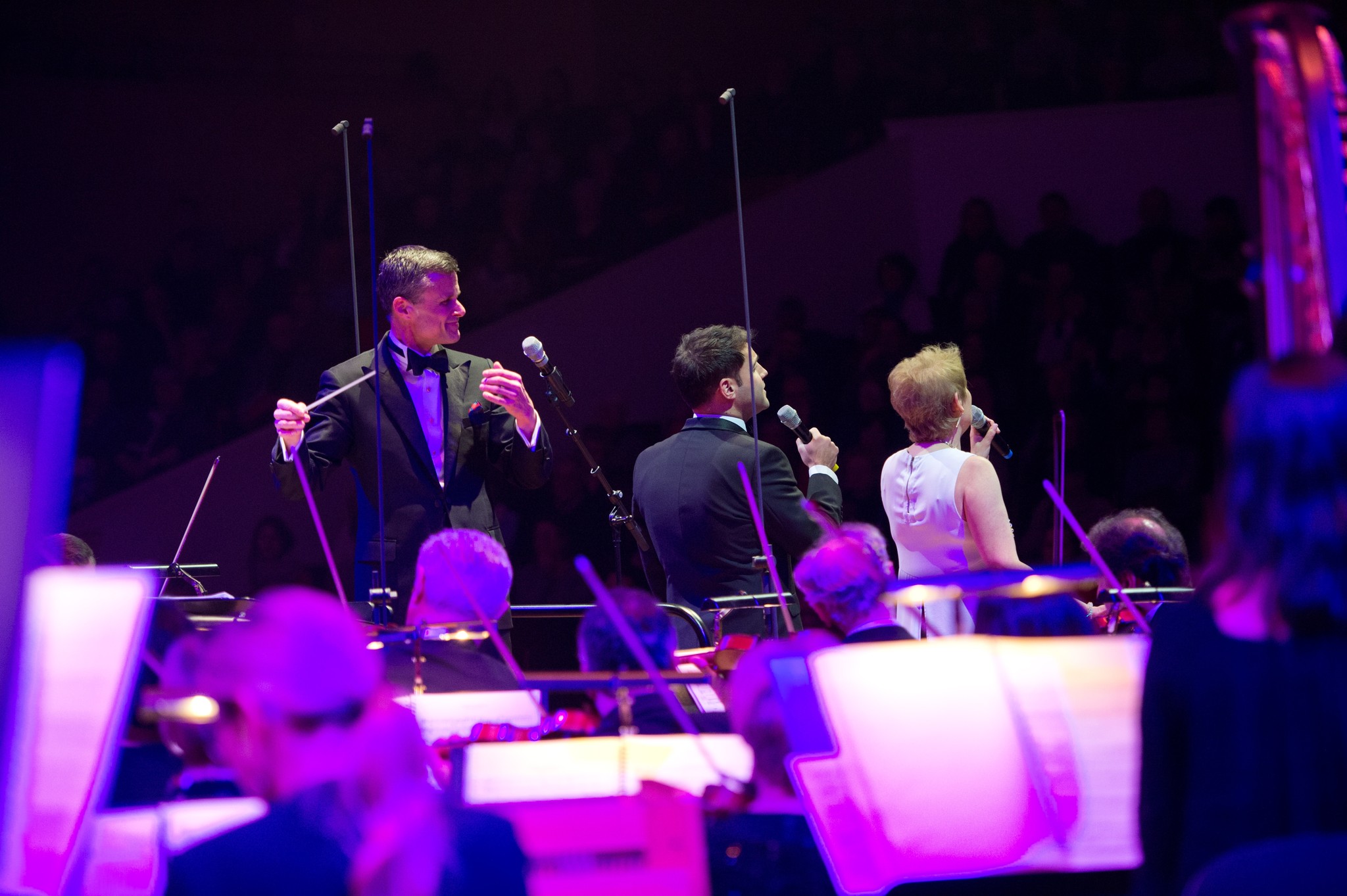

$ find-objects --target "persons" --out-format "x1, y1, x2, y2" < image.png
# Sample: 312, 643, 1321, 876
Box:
788, 521, 916, 642
578, 587, 730, 734
1075, 507, 1194, 613
270, 243, 554, 663
40, 530, 97, 570
159, 582, 533, 896
878, 340, 1098, 639
1136, 347, 1347, 896
381, 527, 524, 695
629, 324, 842, 630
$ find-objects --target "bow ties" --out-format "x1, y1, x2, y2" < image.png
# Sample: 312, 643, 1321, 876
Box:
401, 347, 450, 377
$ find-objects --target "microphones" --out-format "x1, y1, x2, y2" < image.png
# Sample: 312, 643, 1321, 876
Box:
523, 336, 576, 407
971, 405, 1013, 459
777, 404, 839, 472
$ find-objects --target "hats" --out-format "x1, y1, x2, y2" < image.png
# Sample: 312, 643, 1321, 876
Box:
197, 589, 383, 714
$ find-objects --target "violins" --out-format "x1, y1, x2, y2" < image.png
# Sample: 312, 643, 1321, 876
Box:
1087, 600, 1187, 633
674, 646, 746, 678
428, 708, 599, 758
